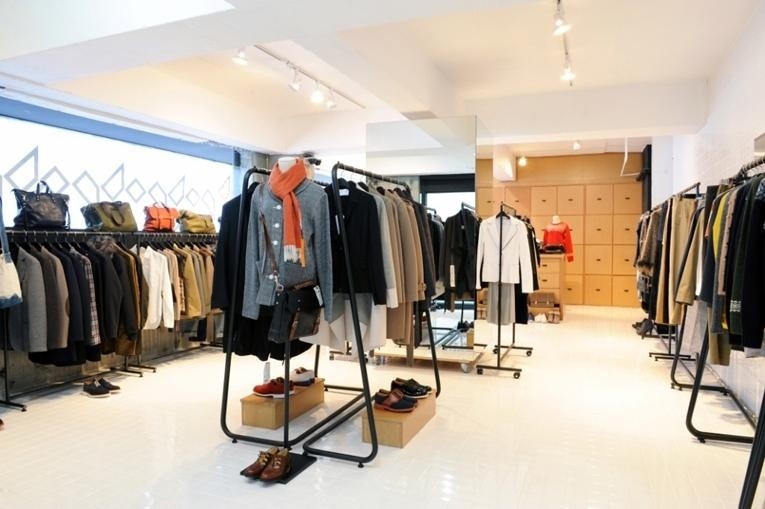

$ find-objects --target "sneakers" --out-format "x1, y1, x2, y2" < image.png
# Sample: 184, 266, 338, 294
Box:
276, 376, 296, 397
377, 387, 420, 406
373, 391, 417, 414
251, 378, 285, 400
97, 377, 122, 394
80, 377, 111, 398
289, 368, 311, 387
298, 365, 315, 384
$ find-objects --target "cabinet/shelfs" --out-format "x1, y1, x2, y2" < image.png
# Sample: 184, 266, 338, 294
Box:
475, 151, 643, 308
477, 251, 565, 320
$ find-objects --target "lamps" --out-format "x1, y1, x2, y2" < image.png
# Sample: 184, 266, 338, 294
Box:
287, 67, 337, 110
519, 156, 527, 165
550, 11, 575, 83
573, 140, 581, 150
232, 47, 249, 66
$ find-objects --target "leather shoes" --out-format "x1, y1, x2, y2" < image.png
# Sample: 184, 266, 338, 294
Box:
259, 447, 293, 482
395, 376, 435, 396
389, 379, 429, 399
239, 445, 280, 481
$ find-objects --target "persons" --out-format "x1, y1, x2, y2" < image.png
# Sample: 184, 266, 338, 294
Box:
241, 157, 333, 322
544, 215, 573, 262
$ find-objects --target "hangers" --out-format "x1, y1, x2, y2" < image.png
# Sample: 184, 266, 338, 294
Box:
737, 154, 764, 179
340, 164, 411, 195
8, 229, 220, 262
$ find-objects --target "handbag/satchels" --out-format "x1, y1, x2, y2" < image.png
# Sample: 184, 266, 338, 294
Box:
10, 179, 72, 233
265, 278, 326, 344
0, 250, 26, 312
77, 199, 140, 232
141, 201, 181, 233
178, 208, 218, 234
